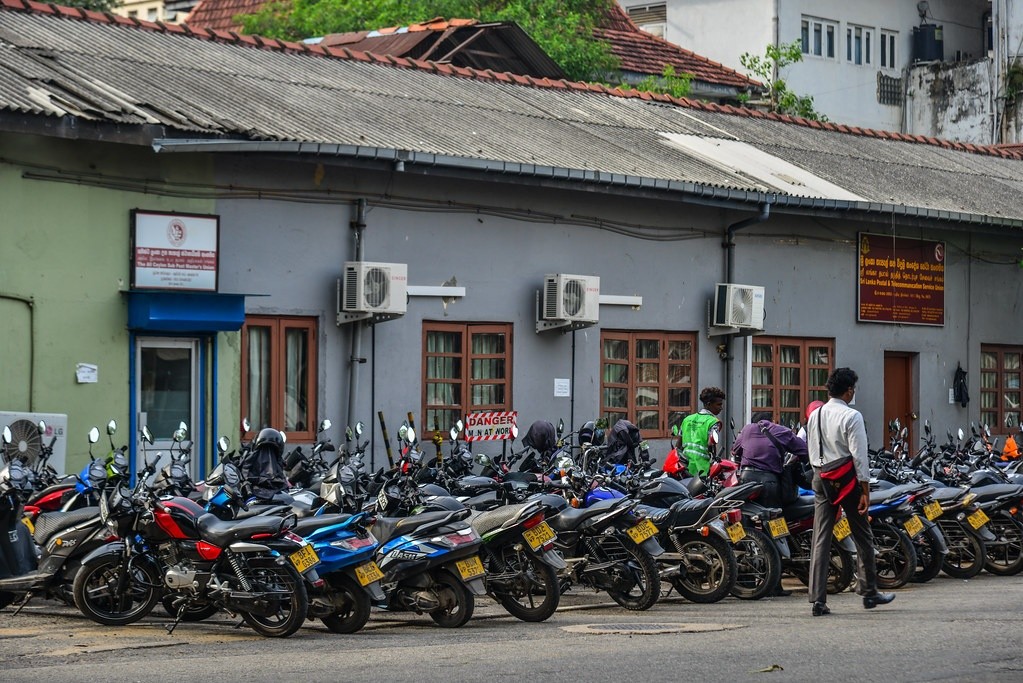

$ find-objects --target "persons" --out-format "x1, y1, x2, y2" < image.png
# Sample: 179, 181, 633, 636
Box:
673, 386, 726, 481
807, 368, 895, 617
731, 400, 825, 597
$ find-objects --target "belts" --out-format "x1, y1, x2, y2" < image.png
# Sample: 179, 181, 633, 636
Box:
743, 466, 765, 471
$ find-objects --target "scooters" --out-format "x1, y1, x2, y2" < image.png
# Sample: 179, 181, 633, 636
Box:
0, 416, 1023, 640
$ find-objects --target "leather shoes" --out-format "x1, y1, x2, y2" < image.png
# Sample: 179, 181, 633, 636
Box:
813, 602, 830, 616
766, 588, 792, 596
863, 593, 895, 609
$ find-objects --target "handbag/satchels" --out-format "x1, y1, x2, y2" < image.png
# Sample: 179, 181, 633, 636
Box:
820, 456, 859, 505
781, 470, 798, 503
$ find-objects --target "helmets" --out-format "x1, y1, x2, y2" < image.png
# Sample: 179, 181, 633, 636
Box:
579, 420, 605, 446
806, 400, 825, 422
607, 420, 640, 448
254, 429, 285, 456
522, 421, 556, 451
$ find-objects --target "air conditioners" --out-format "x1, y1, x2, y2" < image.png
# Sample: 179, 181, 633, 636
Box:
713, 283, 765, 329
344, 260, 410, 315
542, 274, 600, 321
0, 411, 68, 478
